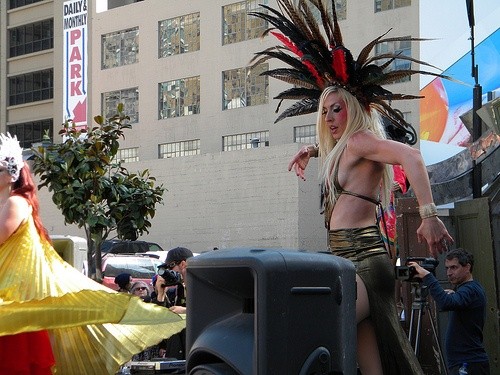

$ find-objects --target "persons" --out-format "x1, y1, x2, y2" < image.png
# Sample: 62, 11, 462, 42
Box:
408, 248, 491, 375
155, 247, 193, 360
114, 273, 134, 293
128, 274, 168, 361
288, 80, 454, 375
0, 131, 187, 375
376, 164, 407, 321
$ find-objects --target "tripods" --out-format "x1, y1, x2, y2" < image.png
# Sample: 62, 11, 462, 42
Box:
407, 286, 450, 375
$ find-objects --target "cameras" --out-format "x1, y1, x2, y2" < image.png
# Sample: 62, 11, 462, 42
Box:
158, 267, 184, 287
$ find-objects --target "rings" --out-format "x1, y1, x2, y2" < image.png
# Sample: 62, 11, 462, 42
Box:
442, 239, 446, 248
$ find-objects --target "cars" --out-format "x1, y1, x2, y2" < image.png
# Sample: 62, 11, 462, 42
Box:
89, 241, 200, 291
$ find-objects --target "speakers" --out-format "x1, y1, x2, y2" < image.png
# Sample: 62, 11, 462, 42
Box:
180, 247, 358, 375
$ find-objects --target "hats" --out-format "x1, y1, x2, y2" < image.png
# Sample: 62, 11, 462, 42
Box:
157, 247, 193, 267
114, 273, 131, 283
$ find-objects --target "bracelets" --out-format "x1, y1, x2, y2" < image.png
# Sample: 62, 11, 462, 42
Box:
308, 142, 319, 158
418, 203, 438, 219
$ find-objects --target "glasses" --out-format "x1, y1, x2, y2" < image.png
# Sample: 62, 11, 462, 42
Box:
165, 262, 181, 269
133, 286, 148, 291
